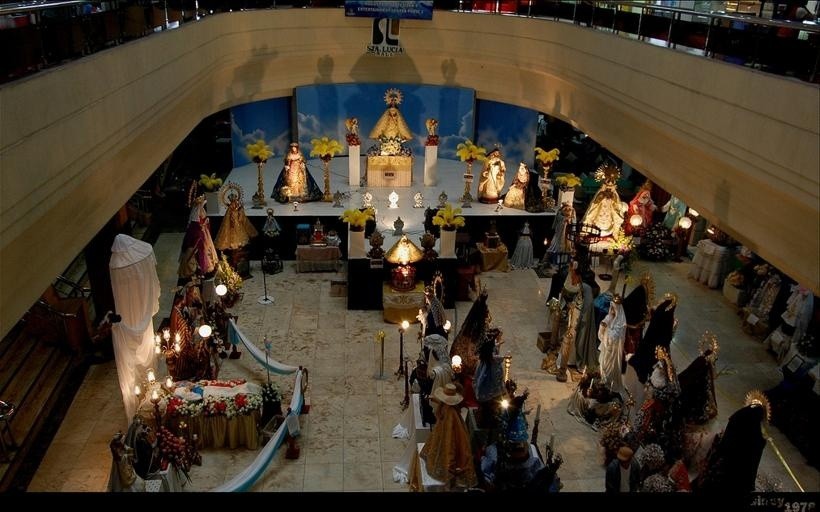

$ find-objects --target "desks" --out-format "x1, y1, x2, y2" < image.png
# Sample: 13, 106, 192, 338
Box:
298, 245, 341, 273
475, 242, 509, 270
382, 283, 427, 324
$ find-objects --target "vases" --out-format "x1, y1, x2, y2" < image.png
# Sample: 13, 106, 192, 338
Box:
347, 228, 366, 261
440, 230, 458, 259
559, 189, 575, 209
207, 192, 221, 214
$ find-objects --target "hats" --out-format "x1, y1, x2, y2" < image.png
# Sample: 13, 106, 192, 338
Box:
433, 382, 465, 407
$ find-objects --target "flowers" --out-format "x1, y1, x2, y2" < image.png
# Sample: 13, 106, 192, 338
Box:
558, 172, 582, 189
339, 206, 378, 230
533, 145, 561, 164
308, 135, 344, 161
198, 172, 223, 189
248, 139, 275, 163
458, 140, 490, 167
431, 202, 466, 228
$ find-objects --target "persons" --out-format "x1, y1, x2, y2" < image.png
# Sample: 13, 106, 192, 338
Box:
421, 283, 564, 491
271, 142, 323, 205
504, 160, 530, 210
159, 181, 260, 377
477, 146, 506, 205
544, 176, 814, 491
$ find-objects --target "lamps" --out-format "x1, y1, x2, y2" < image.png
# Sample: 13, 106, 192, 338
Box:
133, 326, 182, 425
381, 234, 426, 291
